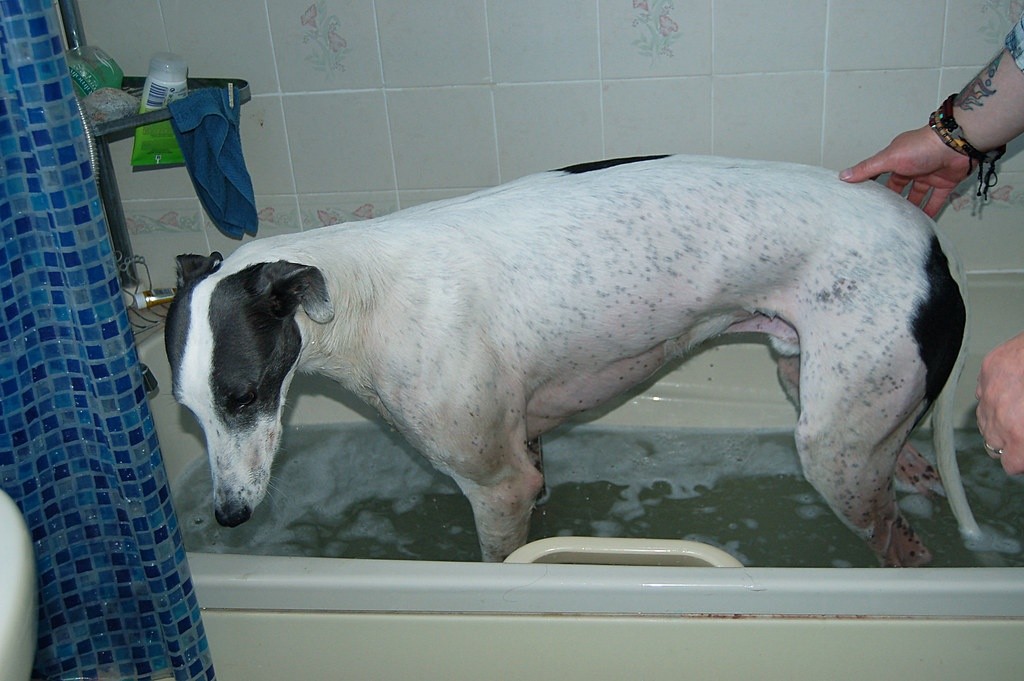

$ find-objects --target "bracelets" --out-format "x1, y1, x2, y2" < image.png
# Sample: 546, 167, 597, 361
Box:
928, 91, 1007, 201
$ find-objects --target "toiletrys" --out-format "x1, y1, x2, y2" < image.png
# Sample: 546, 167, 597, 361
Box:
130, 51, 189, 170
133, 287, 176, 310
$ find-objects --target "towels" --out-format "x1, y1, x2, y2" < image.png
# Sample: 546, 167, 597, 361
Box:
168, 82, 260, 242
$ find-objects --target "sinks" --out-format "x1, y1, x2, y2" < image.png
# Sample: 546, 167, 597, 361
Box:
0, 490, 35, 681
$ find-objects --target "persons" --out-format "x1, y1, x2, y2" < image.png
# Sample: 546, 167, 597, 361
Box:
838, 12, 1024, 474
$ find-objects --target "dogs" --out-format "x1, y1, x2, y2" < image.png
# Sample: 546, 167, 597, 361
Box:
163, 153, 979, 568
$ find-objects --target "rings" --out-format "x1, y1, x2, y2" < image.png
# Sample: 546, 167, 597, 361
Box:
984, 441, 1003, 455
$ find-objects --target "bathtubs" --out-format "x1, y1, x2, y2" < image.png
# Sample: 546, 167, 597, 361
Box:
137, 265, 1024, 679
